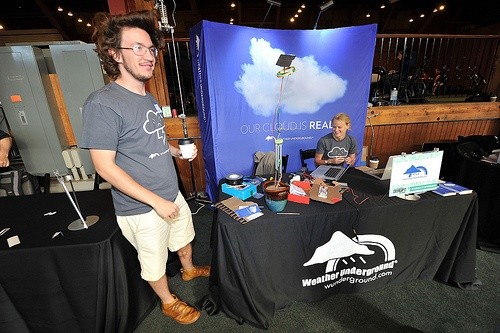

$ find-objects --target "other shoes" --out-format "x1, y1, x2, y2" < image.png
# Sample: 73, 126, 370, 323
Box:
181, 265, 211, 281
161, 294, 200, 324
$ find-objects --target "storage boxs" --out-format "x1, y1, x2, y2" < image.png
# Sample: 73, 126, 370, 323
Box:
221, 182, 256, 200
288, 179, 342, 205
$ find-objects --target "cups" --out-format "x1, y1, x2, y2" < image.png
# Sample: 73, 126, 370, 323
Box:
369, 155, 379, 169
490, 96, 497, 102
178, 138, 194, 160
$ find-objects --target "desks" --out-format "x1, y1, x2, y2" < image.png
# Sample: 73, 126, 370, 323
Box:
458, 139, 500, 254
200, 164, 477, 329
0, 189, 160, 333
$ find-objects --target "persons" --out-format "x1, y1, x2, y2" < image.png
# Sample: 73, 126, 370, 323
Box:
0, 130, 12, 167
77, 12, 212, 324
388, 50, 444, 98
314, 113, 359, 166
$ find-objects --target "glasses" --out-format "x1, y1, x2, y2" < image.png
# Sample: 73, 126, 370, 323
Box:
120, 43, 159, 58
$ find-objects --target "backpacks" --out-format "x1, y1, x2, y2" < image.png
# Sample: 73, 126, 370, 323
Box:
0, 166, 41, 196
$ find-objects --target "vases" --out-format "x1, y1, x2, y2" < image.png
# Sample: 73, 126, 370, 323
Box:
263, 180, 290, 212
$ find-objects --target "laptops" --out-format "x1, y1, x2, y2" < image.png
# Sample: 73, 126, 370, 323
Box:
309, 156, 357, 181
363, 157, 394, 180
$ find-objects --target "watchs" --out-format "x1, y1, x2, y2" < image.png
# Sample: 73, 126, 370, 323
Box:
324, 160, 328, 164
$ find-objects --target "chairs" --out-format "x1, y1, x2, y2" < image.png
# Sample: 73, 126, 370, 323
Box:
421, 136, 498, 191
254, 149, 316, 174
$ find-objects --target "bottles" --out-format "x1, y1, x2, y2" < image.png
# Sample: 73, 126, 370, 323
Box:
389, 87, 398, 105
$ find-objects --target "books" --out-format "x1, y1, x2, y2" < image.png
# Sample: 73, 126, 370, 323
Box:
431, 181, 473, 197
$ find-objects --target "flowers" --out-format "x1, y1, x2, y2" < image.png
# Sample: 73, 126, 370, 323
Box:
275, 139, 283, 189
